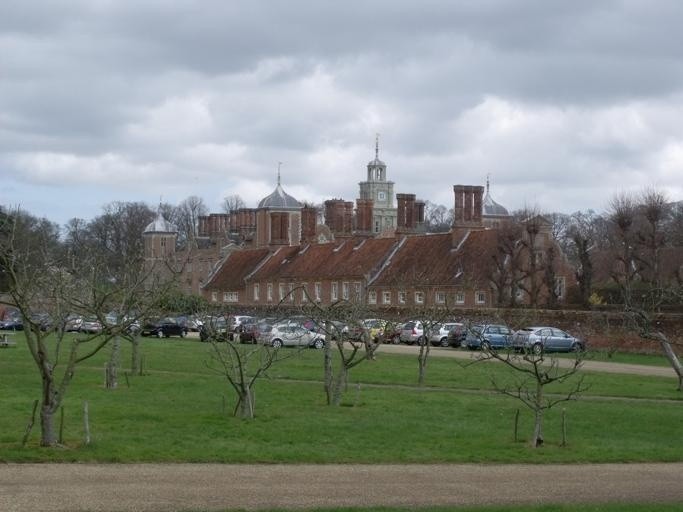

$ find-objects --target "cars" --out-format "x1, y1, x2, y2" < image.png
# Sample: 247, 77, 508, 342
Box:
512, 327, 585, 355
427, 323, 470, 347
216, 315, 278, 345
190, 315, 218, 332
465, 324, 516, 353
257, 323, 326, 349
0, 311, 141, 335
142, 317, 188, 339
400, 321, 441, 347
273, 315, 403, 345
